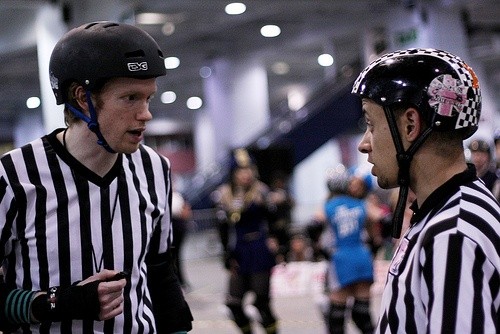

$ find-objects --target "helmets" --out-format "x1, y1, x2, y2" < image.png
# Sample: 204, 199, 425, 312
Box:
347, 165, 371, 200
49, 20, 166, 105
350, 47, 483, 141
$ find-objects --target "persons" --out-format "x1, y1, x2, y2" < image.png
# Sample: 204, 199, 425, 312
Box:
170, 176, 193, 292
351, 48, 500, 334
0, 20, 195, 334
205, 147, 416, 334
468, 128, 500, 200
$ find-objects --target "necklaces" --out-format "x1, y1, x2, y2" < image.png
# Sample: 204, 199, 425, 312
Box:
63, 126, 121, 273
